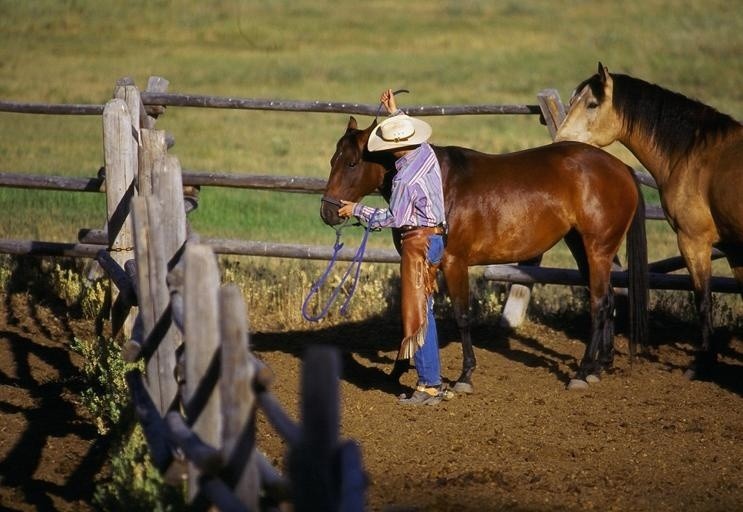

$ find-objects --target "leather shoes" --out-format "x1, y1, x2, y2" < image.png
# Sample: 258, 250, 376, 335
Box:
397, 386, 443, 406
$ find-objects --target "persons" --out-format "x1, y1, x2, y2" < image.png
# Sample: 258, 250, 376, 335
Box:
337, 87, 447, 406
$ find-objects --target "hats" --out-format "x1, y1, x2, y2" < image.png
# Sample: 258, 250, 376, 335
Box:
367, 115, 434, 152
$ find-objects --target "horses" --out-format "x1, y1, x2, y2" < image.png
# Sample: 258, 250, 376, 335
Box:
319, 115, 652, 395
552, 61, 743, 384
